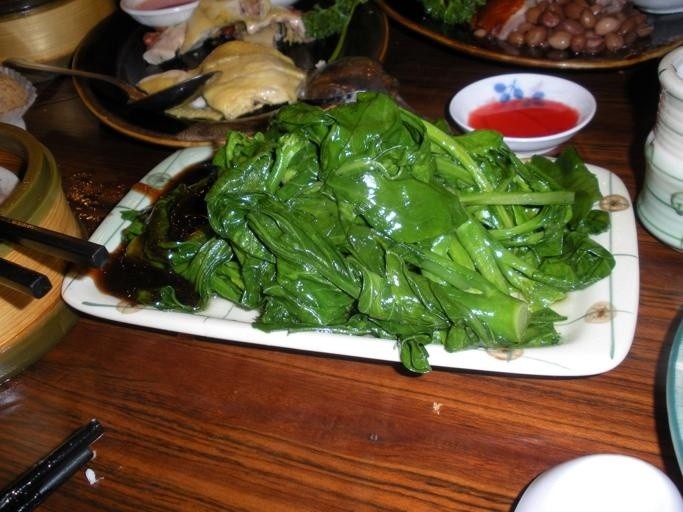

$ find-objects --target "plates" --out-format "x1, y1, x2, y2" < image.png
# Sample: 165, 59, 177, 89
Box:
70, 1, 388, 150
121, 1, 201, 28
449, 72, 599, 161
376, 0, 682, 70
60, 144, 645, 379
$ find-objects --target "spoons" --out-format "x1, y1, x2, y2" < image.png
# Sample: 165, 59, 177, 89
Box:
3, 54, 224, 120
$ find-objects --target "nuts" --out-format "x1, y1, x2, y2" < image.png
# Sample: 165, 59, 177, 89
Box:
472, 0, 657, 53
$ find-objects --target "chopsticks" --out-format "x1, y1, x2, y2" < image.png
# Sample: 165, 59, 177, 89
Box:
1, 215, 108, 302
1, 414, 105, 509
